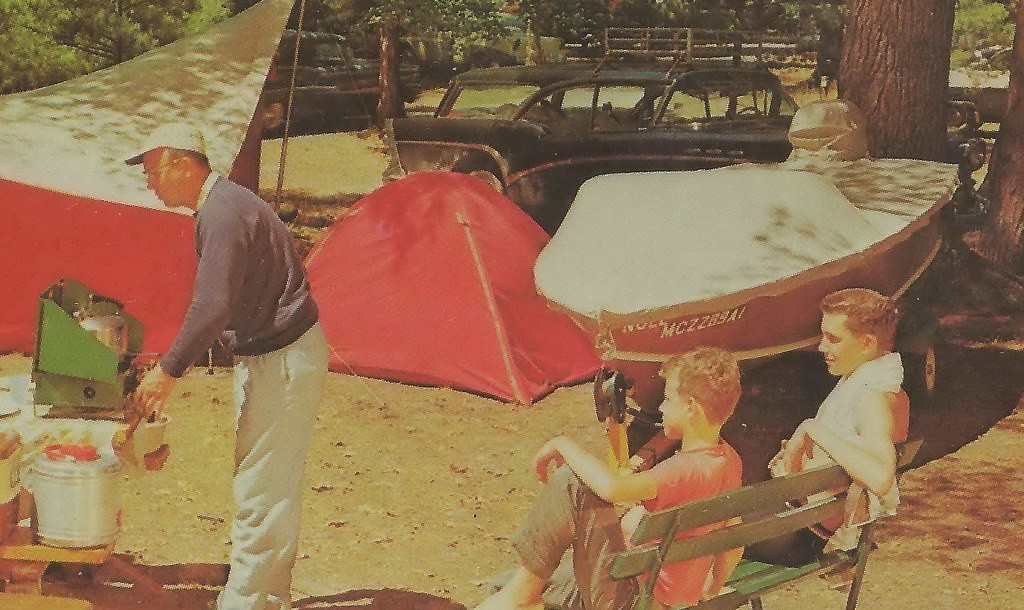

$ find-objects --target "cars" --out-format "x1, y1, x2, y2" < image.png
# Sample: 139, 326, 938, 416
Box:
949, 41, 1012, 96
388, 54, 987, 219
259, 30, 420, 136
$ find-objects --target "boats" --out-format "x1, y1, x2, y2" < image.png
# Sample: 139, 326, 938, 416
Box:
531, 100, 961, 416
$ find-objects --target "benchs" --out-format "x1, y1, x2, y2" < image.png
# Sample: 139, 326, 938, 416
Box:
611, 438, 924, 609
0, 509, 122, 610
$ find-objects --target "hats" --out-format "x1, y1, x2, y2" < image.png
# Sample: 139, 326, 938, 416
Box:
125, 120, 207, 165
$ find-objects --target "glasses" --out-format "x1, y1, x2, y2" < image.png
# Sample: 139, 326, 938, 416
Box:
142, 157, 185, 183
